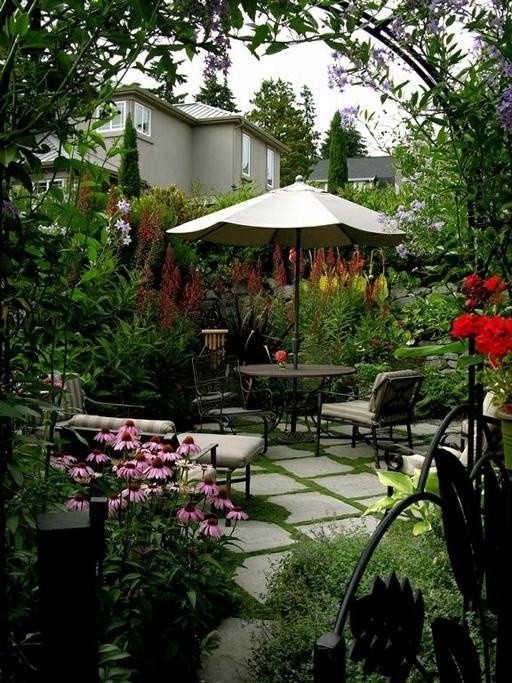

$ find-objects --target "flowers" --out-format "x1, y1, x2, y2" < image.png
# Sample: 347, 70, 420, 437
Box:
449, 270, 512, 409
274, 349, 288, 362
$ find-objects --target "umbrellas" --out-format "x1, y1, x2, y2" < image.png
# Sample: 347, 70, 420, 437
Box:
163, 173, 414, 430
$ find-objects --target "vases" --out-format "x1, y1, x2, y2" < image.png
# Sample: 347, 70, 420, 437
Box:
277, 359, 288, 368
494, 406, 512, 471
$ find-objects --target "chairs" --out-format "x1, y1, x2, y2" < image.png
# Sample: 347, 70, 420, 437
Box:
188, 353, 278, 437
48, 371, 145, 444
50, 410, 269, 505
313, 367, 427, 469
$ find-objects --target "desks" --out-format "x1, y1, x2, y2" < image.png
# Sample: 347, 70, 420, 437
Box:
234, 362, 357, 442
